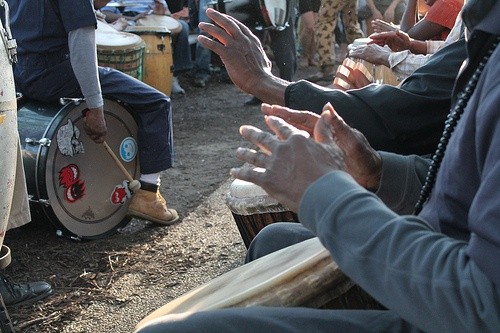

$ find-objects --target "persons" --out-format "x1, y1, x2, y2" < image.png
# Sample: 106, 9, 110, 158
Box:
107, 0, 192, 97
131, 0, 500, 332
195, 0, 500, 159
1, 1, 181, 225
1, 0, 52, 306
293, 0, 465, 79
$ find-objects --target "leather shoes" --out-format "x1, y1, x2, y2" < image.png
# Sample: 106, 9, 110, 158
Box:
0, 275, 53, 308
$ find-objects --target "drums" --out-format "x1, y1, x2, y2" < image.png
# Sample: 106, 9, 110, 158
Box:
225, 166, 301, 250
122, 26, 171, 98
213, 0, 292, 32
134, 235, 390, 333
97, 31, 147, 82
17, 95, 141, 241
136, 15, 183, 47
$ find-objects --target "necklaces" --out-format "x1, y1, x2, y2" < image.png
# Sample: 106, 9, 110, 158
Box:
411, 42, 498, 214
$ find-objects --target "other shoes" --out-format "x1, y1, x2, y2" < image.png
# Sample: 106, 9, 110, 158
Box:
127, 179, 179, 224
171, 75, 185, 95
195, 72, 209, 86
308, 63, 335, 81
245, 96, 263, 104
